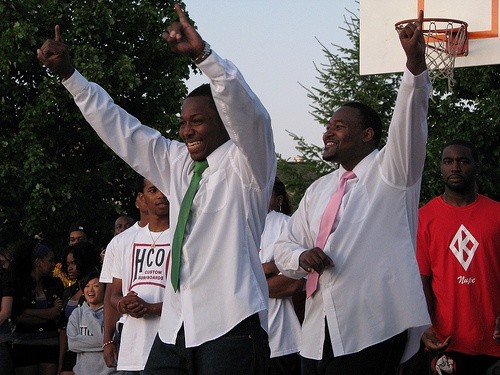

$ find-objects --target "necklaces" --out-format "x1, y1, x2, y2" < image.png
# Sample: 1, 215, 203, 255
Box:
443, 191, 446, 201
149, 227, 164, 249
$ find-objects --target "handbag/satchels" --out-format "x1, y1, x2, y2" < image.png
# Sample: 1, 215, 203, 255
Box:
12, 320, 58, 339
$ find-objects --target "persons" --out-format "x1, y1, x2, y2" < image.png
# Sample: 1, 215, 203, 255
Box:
258, 176, 305, 375
0, 175, 172, 375
273, 10, 433, 375
415, 139, 500, 375
36, 3, 278, 375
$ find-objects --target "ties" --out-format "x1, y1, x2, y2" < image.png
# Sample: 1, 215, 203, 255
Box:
306, 171, 357, 299
171, 161, 208, 293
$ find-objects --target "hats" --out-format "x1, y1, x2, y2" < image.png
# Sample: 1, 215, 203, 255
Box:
69, 220, 91, 236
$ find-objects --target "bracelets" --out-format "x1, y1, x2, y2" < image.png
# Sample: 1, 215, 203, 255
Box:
102, 341, 116, 349
117, 300, 124, 317
